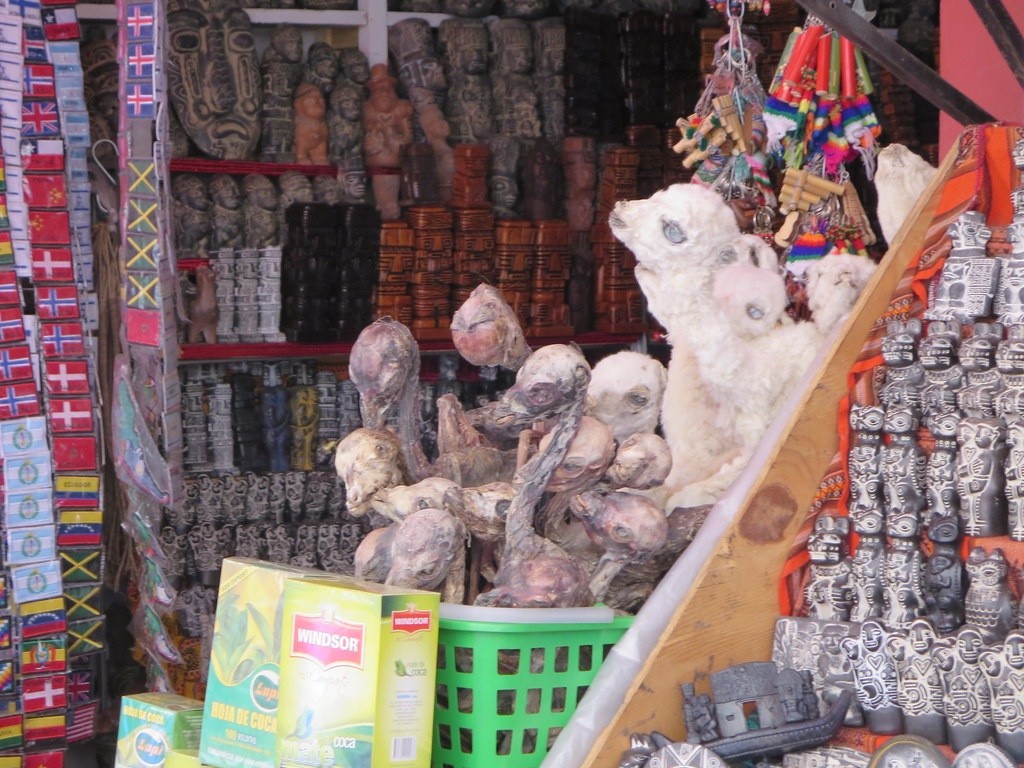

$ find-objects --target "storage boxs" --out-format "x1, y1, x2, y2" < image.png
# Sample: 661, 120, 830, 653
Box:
198, 555, 352, 768
116, 690, 205, 767
277, 577, 442, 766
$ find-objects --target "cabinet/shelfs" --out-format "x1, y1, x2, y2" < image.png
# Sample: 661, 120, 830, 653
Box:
57, 0, 942, 767
530, 125, 1024, 768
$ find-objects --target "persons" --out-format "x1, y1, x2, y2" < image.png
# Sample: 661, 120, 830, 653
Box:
156, 0, 704, 477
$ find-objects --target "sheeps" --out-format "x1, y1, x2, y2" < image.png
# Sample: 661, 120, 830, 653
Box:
334, 142, 940, 606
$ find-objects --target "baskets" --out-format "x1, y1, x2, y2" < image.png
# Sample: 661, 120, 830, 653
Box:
428, 601, 638, 768
